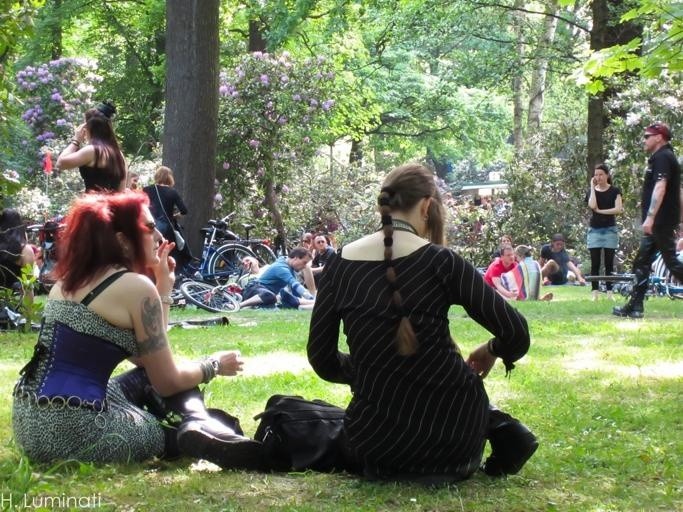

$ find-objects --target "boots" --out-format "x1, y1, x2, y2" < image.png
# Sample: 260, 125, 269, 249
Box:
613, 291, 644, 317
480, 411, 538, 478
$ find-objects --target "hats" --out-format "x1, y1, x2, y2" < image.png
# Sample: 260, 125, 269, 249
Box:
644, 120, 672, 141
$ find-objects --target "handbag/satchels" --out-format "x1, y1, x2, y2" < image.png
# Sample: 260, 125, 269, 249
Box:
253, 394, 346, 474
174, 230, 185, 252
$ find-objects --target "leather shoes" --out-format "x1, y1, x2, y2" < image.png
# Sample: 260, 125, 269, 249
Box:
176, 417, 264, 470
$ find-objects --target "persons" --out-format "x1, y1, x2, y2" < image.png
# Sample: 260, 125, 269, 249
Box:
541, 233, 586, 286
586, 163, 622, 302
306, 163, 538, 486
483, 234, 553, 303
651, 223, 683, 297
612, 122, 683, 318
236, 227, 336, 311
56, 100, 128, 193
126, 172, 138, 190
12, 189, 263, 469
143, 166, 188, 290
0, 208, 34, 333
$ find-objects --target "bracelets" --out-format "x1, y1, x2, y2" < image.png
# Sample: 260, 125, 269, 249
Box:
71, 139, 80, 146
198, 360, 215, 384
160, 295, 174, 304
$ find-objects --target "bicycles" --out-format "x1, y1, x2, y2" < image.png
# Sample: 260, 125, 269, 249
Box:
584, 249, 683, 302
164, 213, 277, 316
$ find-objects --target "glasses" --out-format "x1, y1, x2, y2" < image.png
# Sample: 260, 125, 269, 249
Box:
644, 133, 658, 139
146, 220, 156, 233
